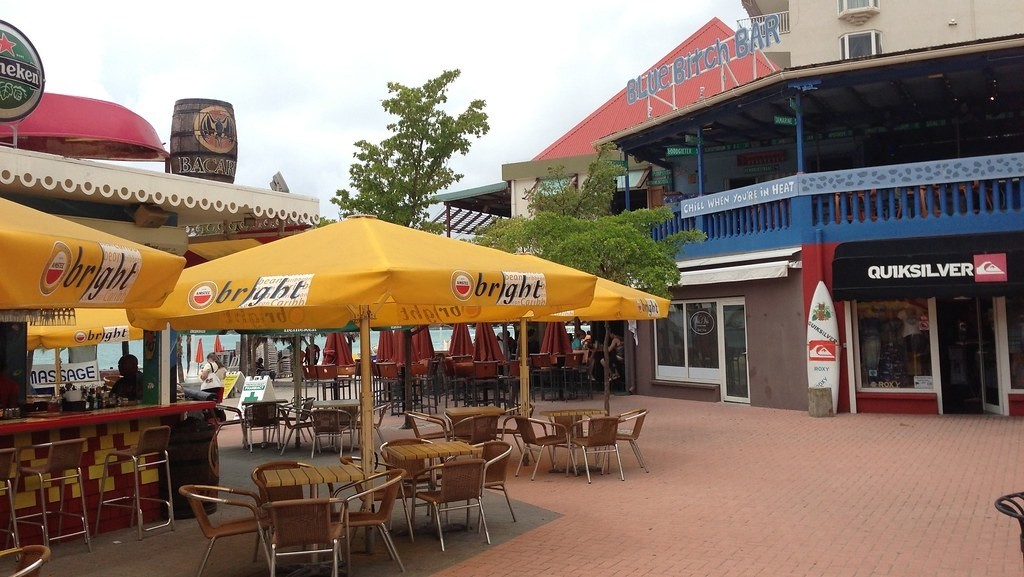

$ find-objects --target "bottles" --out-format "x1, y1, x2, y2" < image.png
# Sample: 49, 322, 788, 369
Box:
98, 396, 102, 408
101, 390, 104, 396
92, 394, 98, 408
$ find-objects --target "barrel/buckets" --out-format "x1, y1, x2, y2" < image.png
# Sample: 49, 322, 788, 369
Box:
170, 98, 238, 184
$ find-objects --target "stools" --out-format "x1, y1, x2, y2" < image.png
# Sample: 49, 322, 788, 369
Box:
94, 425, 176, 539
6, 437, 93, 561
0, 448, 22, 562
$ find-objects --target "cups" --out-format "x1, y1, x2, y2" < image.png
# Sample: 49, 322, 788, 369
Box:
66, 390, 81, 401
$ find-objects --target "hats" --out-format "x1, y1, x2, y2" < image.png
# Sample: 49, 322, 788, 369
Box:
498, 333, 503, 340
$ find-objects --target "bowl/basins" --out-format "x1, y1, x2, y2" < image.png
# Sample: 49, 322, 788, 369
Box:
62, 399, 86, 411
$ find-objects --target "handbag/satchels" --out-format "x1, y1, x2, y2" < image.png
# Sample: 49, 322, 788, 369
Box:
200, 372, 224, 393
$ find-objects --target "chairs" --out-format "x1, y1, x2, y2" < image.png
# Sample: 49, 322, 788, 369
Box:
340, 456, 414, 548
261, 497, 348, 576
515, 415, 577, 480
253, 460, 313, 562
214, 395, 391, 456
405, 410, 452, 440
596, 408, 649, 474
301, 349, 594, 417
179, 485, 271, 576
412, 459, 491, 551
453, 413, 500, 444
380, 437, 450, 525
331, 468, 405, 576
0, 544, 51, 576
446, 440, 517, 533
566, 415, 625, 484
994, 491, 1024, 561
495, 403, 535, 462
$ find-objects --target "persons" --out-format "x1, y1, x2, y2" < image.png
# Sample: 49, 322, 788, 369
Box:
572, 331, 595, 381
291, 338, 320, 382
110, 355, 144, 402
496, 329, 538, 355
600, 331, 624, 381
199, 353, 227, 421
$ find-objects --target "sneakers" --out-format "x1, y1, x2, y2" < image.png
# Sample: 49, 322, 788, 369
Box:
588, 374, 596, 381
609, 372, 620, 382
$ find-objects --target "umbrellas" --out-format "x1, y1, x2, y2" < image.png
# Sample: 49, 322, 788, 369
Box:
0, 196, 674, 577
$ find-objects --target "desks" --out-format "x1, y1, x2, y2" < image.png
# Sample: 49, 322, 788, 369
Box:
309, 399, 360, 452
538, 409, 608, 466
385, 440, 481, 525
262, 464, 367, 576
446, 406, 505, 416
242, 398, 289, 453
